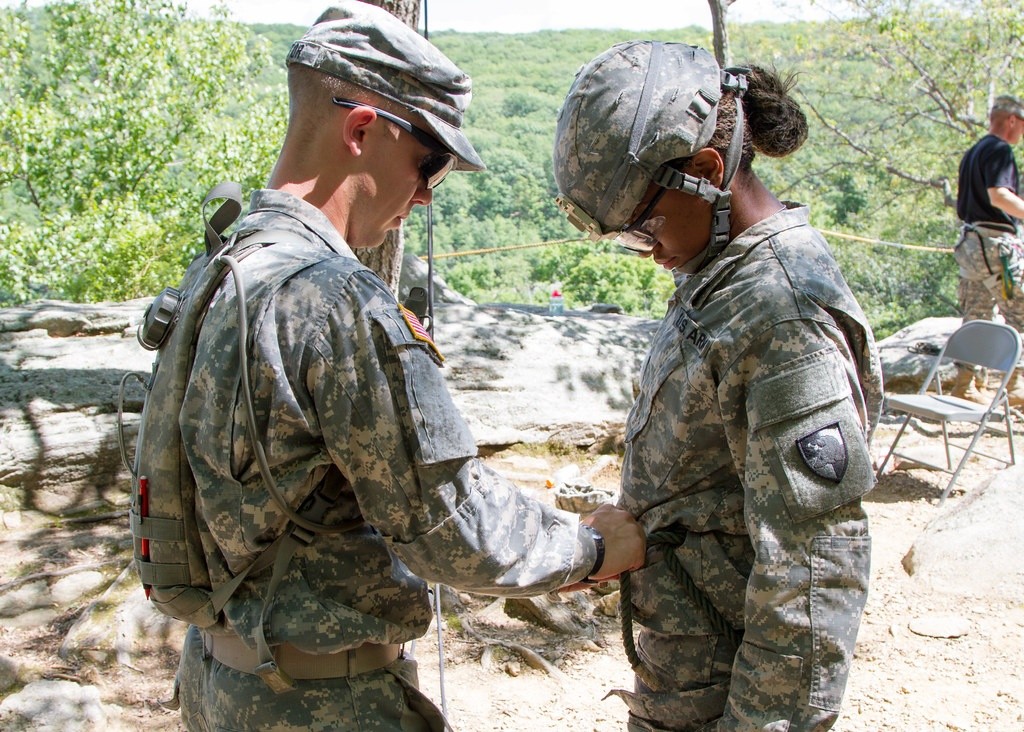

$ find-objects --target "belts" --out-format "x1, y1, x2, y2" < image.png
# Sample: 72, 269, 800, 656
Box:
191, 625, 406, 678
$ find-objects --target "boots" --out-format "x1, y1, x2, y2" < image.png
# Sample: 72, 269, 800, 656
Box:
1000, 369, 1024, 404
949, 368, 993, 406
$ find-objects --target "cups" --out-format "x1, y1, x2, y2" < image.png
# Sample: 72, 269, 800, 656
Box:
551, 463, 582, 481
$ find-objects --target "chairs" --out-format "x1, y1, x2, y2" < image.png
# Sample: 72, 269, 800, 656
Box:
878, 319, 1022, 506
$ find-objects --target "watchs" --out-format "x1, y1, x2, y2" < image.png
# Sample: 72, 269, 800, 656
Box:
580, 525, 605, 584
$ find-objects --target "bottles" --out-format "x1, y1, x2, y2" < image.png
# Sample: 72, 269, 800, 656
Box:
549, 291, 563, 316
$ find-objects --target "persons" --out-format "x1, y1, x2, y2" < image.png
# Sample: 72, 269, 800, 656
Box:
552, 40, 885, 731
173, 0, 648, 732
953, 96, 1024, 406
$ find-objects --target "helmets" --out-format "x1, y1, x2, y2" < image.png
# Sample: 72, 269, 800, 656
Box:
553, 41, 721, 241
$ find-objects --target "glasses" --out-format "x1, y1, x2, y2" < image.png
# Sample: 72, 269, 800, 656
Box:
613, 158, 702, 253
332, 96, 458, 190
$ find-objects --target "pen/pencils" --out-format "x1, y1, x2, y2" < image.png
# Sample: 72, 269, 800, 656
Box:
138, 476, 153, 601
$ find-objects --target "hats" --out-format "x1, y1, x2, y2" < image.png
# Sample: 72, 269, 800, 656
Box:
994, 95, 1024, 119
286, 0, 487, 170
